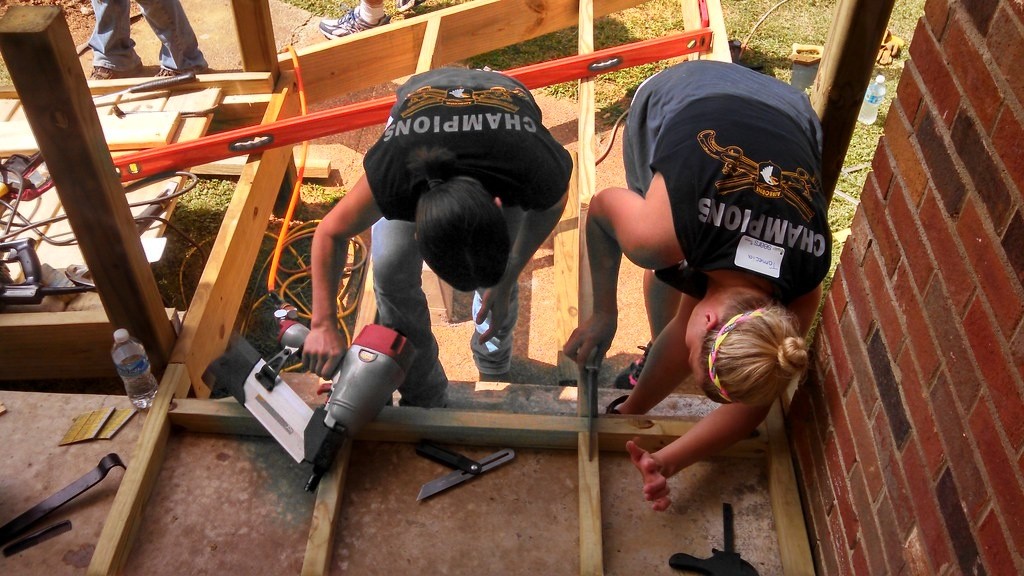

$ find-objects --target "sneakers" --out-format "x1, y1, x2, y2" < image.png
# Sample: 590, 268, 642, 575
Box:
395, 0, 427, 12
604, 395, 629, 415
88, 61, 143, 78
318, 4, 392, 41
614, 340, 653, 390
154, 65, 209, 76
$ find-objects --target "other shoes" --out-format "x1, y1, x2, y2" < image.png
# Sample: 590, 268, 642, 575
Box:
399, 399, 448, 410
480, 371, 514, 384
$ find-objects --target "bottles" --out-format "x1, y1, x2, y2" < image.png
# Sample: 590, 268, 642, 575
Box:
110, 329, 159, 409
857, 75, 887, 126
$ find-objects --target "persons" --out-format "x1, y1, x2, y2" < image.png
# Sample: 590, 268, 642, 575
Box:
302, 67, 575, 409
81, 0, 210, 90
317, 1, 426, 39
563, 57, 833, 514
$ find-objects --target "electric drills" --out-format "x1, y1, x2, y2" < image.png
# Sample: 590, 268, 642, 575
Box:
200, 290, 418, 494
